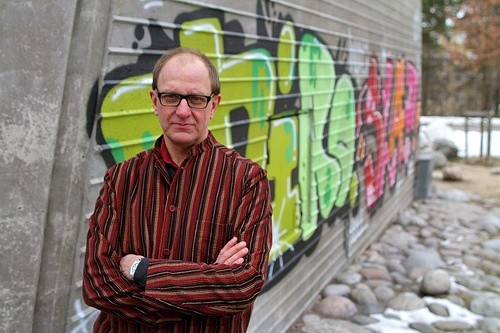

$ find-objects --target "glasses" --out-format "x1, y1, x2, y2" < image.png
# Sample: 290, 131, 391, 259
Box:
156, 88, 215, 109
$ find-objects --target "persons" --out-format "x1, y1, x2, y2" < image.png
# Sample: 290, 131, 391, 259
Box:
82, 46, 272, 333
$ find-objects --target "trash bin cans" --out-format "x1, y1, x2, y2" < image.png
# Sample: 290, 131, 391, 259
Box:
415, 156, 432, 199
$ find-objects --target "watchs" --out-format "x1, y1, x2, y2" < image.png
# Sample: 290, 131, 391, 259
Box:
129, 260, 144, 279
